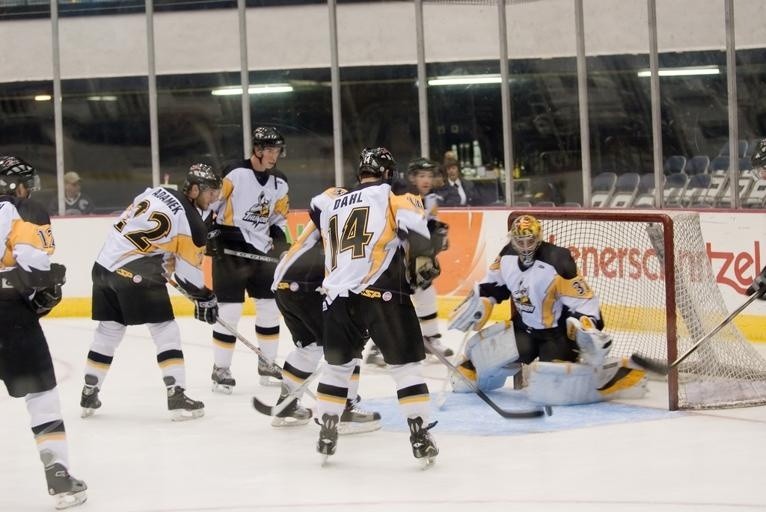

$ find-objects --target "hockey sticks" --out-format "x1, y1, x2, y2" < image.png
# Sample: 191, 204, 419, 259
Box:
424, 335, 553, 420
631, 292, 761, 374
439, 326, 473, 407
254, 362, 322, 416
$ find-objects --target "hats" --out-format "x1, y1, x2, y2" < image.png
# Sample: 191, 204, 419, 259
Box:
64, 172, 81, 182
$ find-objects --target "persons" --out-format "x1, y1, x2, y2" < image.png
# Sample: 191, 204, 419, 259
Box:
272, 221, 383, 432
447, 215, 668, 405
81, 166, 219, 411
366, 157, 453, 366
0, 156, 89, 497
436, 158, 488, 207
309, 147, 448, 467
48, 172, 93, 217
201, 126, 292, 387
747, 138, 766, 301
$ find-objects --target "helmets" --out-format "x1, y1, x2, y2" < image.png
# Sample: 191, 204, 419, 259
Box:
407, 157, 435, 175
356, 147, 396, 184
0, 154, 41, 199
510, 214, 542, 266
186, 162, 226, 190
253, 125, 285, 148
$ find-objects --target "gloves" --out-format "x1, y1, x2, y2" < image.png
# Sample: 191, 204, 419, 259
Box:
204, 229, 224, 258
32, 289, 64, 317
191, 295, 220, 325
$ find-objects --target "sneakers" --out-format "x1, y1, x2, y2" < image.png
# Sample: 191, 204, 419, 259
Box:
45, 463, 88, 497
79, 386, 102, 409
366, 345, 385, 367
167, 384, 204, 412
257, 358, 282, 379
276, 395, 380, 454
212, 363, 236, 385
423, 338, 452, 356
410, 432, 439, 458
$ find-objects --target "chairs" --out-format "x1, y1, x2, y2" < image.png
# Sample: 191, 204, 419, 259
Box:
487, 138, 766, 209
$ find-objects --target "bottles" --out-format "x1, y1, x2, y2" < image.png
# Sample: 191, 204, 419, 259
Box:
452, 141, 525, 180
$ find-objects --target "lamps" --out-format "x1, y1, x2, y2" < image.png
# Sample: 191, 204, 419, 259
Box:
415, 74, 515, 87
210, 82, 295, 96
638, 65, 720, 78
